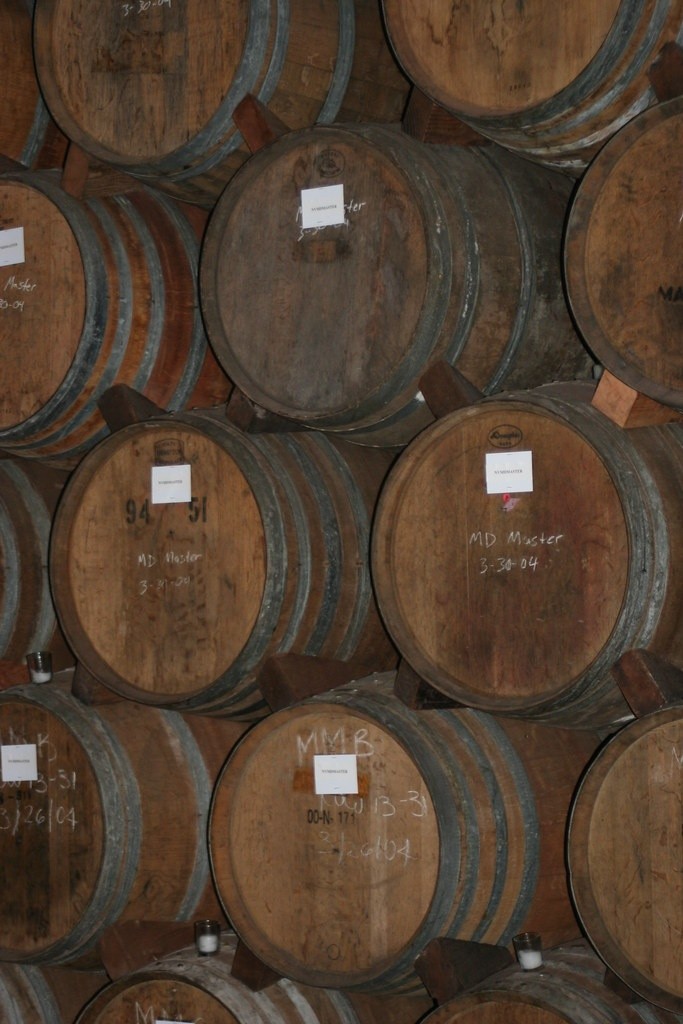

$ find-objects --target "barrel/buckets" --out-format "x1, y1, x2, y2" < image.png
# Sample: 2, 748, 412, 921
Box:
206, 128, 579, 452
420, 937, 683, 1024
0, 673, 256, 968
568, 705, 683, 1014
563, 95, 683, 414
0, 0, 119, 178
369, 377, 683, 735
0, 457, 77, 676
0, 169, 233, 472
207, 668, 610, 997
48, 405, 401, 717
33, 0, 412, 210
382, 0, 683, 180
70, 927, 437, 1024
0, 960, 104, 1024
